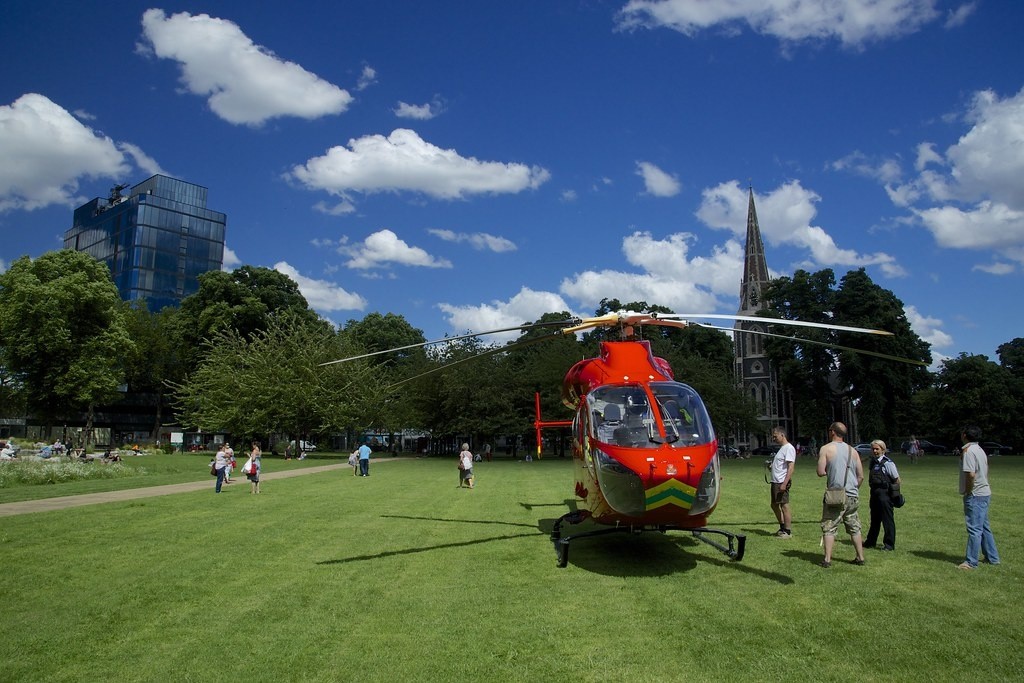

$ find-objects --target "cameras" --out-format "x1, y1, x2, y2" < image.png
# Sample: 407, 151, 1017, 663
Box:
765, 459, 772, 467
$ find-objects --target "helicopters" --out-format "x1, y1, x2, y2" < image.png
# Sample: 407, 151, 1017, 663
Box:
314, 307, 930, 569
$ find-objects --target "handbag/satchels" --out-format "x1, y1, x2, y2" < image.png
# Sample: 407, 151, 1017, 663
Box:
240, 457, 252, 474
210, 463, 216, 476
822, 488, 846, 508
464, 474, 474, 485
248, 461, 257, 476
458, 461, 464, 470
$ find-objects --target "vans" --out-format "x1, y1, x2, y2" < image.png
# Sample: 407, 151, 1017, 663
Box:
290, 440, 316, 452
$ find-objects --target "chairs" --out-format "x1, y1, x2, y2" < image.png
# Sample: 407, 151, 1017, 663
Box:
597, 404, 628, 444
661, 400, 691, 434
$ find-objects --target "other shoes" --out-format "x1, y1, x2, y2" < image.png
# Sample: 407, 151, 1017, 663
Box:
770, 530, 783, 537
881, 545, 895, 552
849, 558, 865, 566
819, 559, 832, 568
861, 541, 876, 548
778, 531, 792, 539
957, 563, 972, 569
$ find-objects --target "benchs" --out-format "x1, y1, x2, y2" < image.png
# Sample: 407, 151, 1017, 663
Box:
51, 448, 64, 455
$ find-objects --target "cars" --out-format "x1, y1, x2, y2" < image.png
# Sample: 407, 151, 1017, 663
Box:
718, 445, 741, 456
752, 446, 777, 455
853, 444, 890, 455
978, 441, 1013, 457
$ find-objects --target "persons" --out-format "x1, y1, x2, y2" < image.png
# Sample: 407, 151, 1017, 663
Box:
795, 442, 801, 457
156, 440, 161, 449
422, 448, 427, 456
132, 444, 142, 455
285, 444, 306, 461
959, 425, 1001, 569
348, 441, 373, 477
208, 441, 262, 495
816, 422, 865, 568
474, 443, 492, 462
907, 434, 924, 465
458, 442, 473, 488
382, 442, 397, 456
770, 425, 797, 539
862, 440, 905, 549
526, 455, 533, 462
54, 436, 84, 458
104, 449, 121, 462
0, 444, 14, 461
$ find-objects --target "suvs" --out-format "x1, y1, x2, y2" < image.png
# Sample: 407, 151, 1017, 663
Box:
901, 439, 946, 456
